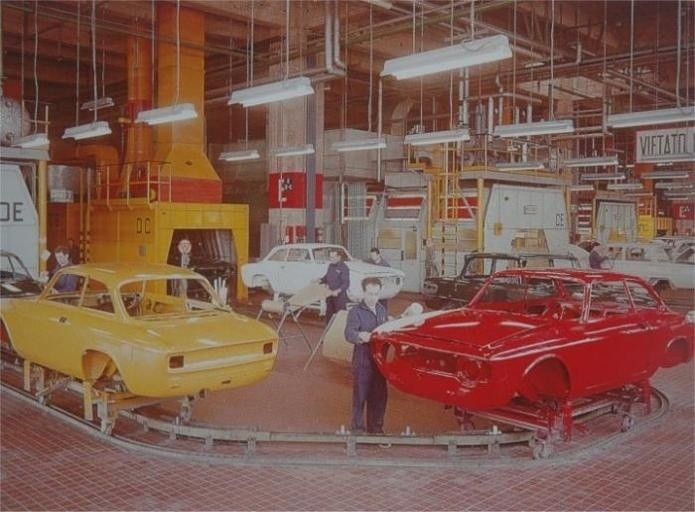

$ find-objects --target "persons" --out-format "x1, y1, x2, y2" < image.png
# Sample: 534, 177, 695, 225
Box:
589, 242, 610, 270
364, 248, 392, 310
167, 239, 200, 299
67, 240, 78, 264
318, 248, 351, 345
39, 245, 81, 294
344, 276, 393, 448
423, 236, 440, 279
